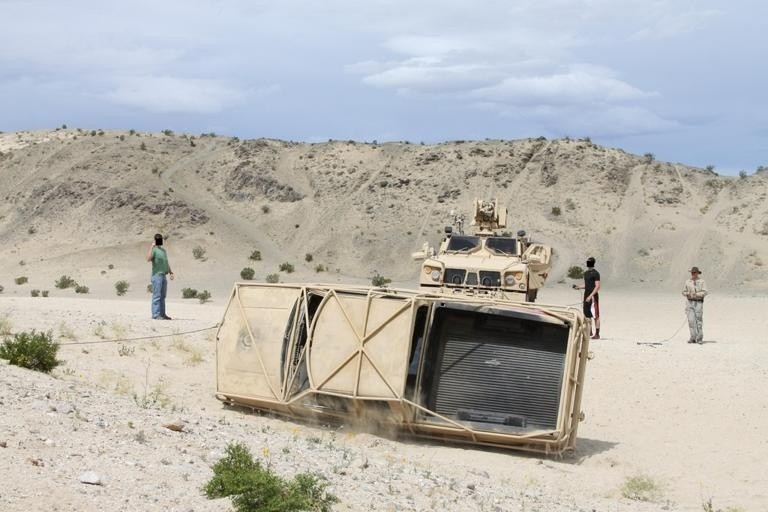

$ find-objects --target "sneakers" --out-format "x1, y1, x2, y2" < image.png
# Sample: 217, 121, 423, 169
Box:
688, 339, 696, 343
697, 338, 703, 345
591, 335, 600, 338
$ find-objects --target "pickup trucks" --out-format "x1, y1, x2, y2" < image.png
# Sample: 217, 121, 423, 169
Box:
212, 278, 594, 463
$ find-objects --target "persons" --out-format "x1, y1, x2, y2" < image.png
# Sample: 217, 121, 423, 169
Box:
680, 265, 709, 345
146, 233, 174, 319
575, 257, 602, 340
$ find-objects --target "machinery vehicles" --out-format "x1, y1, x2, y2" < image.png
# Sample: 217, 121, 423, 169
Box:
412, 199, 554, 304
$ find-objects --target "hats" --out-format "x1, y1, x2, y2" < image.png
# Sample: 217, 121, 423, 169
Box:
688, 266, 702, 274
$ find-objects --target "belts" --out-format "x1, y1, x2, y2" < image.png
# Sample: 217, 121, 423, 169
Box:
687, 298, 702, 302
